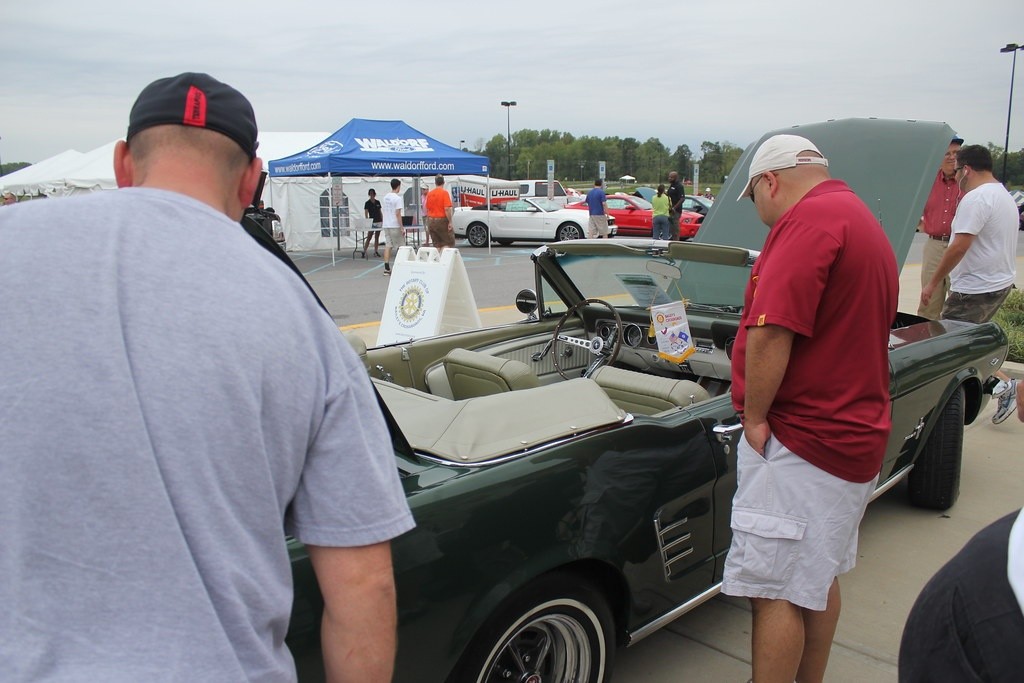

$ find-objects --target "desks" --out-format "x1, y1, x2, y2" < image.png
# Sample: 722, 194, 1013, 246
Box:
348, 224, 432, 260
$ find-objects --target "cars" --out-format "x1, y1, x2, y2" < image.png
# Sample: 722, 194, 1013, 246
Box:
564, 194, 705, 241
285, 115, 1010, 683
451, 196, 619, 248
633, 184, 714, 217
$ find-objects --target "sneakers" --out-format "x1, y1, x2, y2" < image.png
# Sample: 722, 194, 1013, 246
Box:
992, 377, 1023, 424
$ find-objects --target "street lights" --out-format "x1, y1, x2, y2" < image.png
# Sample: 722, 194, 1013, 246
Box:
500, 100, 517, 181
1000, 43, 1024, 187
460, 139, 465, 151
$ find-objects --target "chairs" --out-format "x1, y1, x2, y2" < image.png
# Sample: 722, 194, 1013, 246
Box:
443, 346, 537, 407
589, 364, 710, 426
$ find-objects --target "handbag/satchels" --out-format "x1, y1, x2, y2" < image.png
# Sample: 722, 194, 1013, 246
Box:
668, 197, 676, 224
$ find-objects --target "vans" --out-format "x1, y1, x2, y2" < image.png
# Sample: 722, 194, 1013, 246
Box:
516, 179, 568, 208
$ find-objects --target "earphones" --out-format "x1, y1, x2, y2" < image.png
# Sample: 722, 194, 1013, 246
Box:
964, 170, 967, 176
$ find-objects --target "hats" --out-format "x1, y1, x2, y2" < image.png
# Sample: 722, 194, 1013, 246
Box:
421, 185, 429, 190
128, 73, 259, 164
950, 134, 964, 146
736, 134, 828, 203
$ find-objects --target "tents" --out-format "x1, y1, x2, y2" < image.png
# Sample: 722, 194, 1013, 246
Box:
1, 136, 126, 199
256, 132, 461, 251
619, 175, 635, 184
268, 119, 491, 253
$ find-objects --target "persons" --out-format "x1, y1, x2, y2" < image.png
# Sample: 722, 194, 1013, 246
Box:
917, 138, 964, 318
719, 134, 898, 683
382, 179, 406, 276
921, 145, 1021, 425
705, 188, 714, 200
1, 72, 415, 683
586, 180, 609, 238
4, 193, 16, 204
421, 185, 430, 245
361, 189, 382, 258
666, 172, 685, 240
652, 185, 670, 240
425, 174, 455, 247
896, 379, 1024, 682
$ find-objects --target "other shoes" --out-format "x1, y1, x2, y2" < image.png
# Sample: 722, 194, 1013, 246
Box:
361, 252, 366, 258
374, 252, 381, 257
383, 270, 391, 276
422, 243, 429, 246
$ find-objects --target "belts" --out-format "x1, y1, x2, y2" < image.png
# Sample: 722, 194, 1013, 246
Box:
928, 234, 951, 243
428, 217, 448, 221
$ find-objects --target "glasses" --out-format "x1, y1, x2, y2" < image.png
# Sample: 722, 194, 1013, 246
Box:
945, 150, 957, 158
953, 166, 965, 176
749, 172, 780, 204
